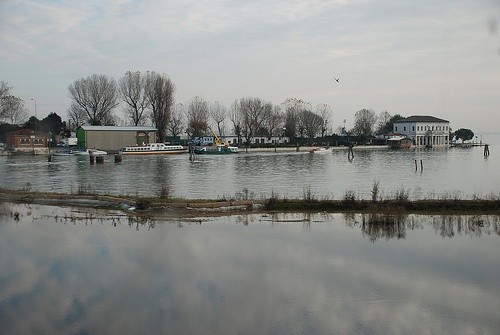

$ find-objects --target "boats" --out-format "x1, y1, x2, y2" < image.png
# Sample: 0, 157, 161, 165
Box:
72, 149, 107, 156
120, 142, 183, 156
196, 144, 238, 153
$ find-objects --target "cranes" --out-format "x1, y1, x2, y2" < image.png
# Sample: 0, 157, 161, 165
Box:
208, 126, 229, 146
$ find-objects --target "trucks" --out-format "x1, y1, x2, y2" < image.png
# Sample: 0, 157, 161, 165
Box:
57, 138, 77, 147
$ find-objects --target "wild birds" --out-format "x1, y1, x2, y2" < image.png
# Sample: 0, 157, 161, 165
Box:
334, 76, 340, 83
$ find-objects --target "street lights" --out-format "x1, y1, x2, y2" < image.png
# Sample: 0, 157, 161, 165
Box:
31, 98, 36, 137
